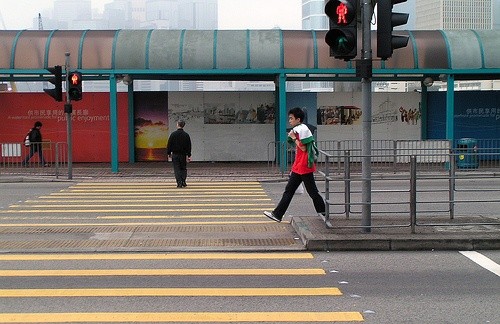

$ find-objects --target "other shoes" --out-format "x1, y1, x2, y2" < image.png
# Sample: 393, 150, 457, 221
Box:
43, 164, 50, 167
183, 181, 187, 186
23, 164, 30, 167
177, 183, 182, 187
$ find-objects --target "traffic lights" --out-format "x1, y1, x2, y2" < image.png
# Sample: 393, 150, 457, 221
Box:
69, 72, 82, 101
324, 0, 360, 62
43, 65, 62, 101
377, 0, 409, 60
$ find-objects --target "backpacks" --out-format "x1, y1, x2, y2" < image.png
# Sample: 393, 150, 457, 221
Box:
23, 128, 34, 147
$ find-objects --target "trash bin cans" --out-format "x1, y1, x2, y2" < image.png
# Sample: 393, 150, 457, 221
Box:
456, 138, 479, 171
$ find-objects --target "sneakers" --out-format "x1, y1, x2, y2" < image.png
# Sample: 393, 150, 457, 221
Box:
263, 211, 281, 223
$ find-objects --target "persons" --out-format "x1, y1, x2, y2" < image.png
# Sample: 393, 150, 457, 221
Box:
326, 108, 339, 118
399, 107, 419, 125
23, 121, 47, 167
263, 108, 325, 223
167, 120, 191, 187
